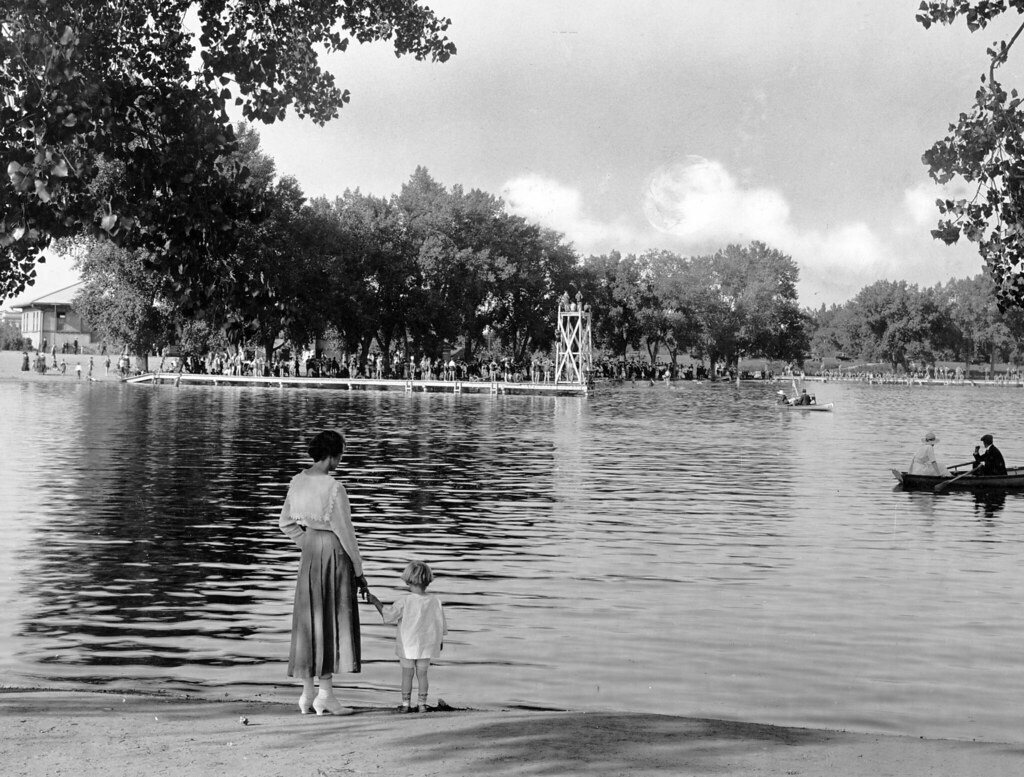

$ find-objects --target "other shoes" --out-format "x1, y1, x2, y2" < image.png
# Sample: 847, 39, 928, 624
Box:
400, 706, 410, 713
417, 704, 427, 713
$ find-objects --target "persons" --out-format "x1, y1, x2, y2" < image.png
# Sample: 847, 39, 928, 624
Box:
42, 337, 47, 352
116, 351, 1024, 386
777, 390, 790, 404
367, 560, 448, 713
972, 435, 1008, 475
101, 341, 108, 355
51, 345, 56, 356
88, 356, 94, 371
795, 388, 811, 407
908, 432, 952, 476
75, 362, 83, 377
22, 351, 66, 374
809, 396, 816, 405
279, 430, 369, 718
105, 356, 111, 371
74, 338, 78, 354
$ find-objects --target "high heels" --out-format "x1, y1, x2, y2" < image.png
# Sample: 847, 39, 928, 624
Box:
299, 695, 313, 713
313, 696, 353, 716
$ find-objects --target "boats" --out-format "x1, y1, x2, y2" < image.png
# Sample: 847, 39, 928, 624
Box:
777, 402, 833, 412
900, 466, 1024, 492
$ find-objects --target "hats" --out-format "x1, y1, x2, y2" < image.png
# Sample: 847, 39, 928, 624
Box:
921, 433, 940, 445
981, 435, 993, 441
777, 390, 785, 395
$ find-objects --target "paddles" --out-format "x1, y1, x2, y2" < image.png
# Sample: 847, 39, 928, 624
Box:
934, 463, 983, 494
901, 460, 976, 475
